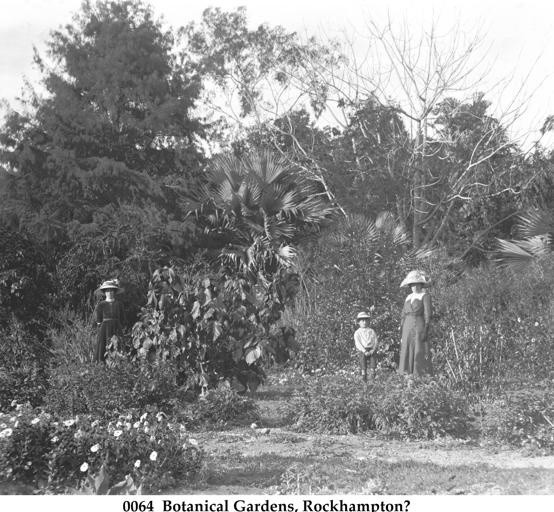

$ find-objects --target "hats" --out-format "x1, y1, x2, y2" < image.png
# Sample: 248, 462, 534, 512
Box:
93, 281, 124, 296
356, 311, 371, 319
399, 270, 431, 288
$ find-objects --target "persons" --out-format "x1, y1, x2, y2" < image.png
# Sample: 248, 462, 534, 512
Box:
397, 271, 432, 377
353, 311, 378, 383
92, 280, 126, 366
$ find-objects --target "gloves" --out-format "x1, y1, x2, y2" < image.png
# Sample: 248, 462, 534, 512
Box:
399, 325, 402, 340
420, 323, 429, 342
